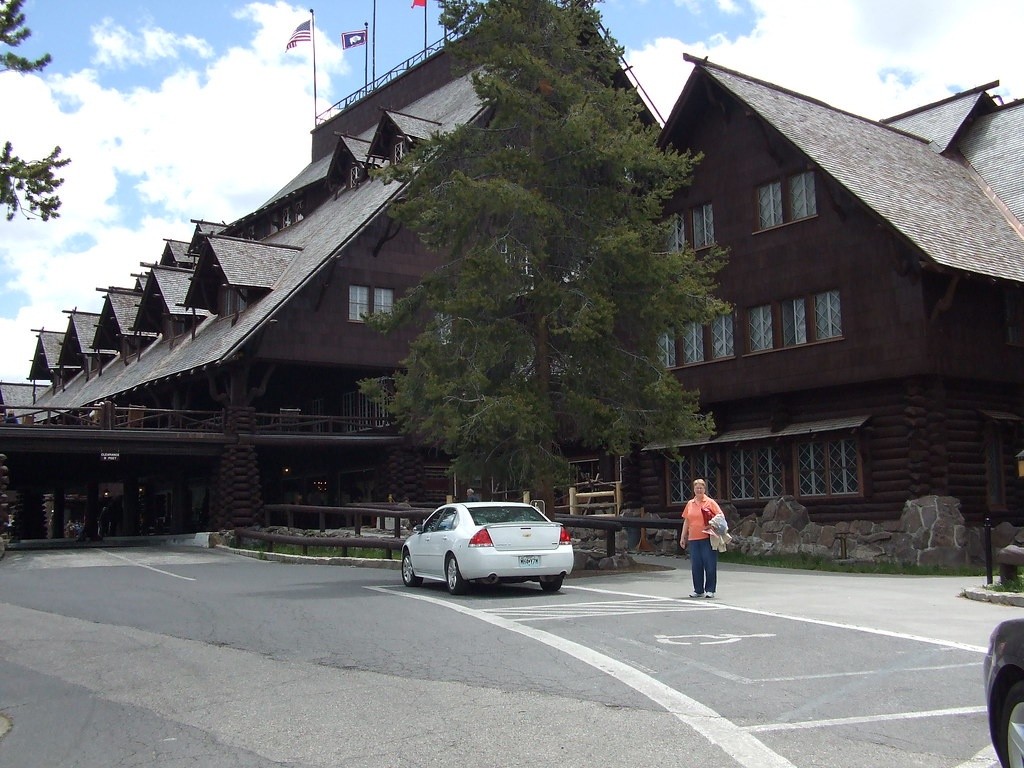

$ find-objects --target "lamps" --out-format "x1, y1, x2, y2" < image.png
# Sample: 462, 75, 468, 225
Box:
1015, 450, 1024, 479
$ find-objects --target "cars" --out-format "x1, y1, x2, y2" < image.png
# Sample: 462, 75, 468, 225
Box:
400, 501, 575, 596
982, 618, 1024, 768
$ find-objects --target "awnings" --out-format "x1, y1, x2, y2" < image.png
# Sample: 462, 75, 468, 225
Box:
641, 412, 872, 451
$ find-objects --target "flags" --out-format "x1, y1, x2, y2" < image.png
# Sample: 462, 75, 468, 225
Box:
411, 0, 426, 9
341, 30, 366, 50
285, 19, 311, 51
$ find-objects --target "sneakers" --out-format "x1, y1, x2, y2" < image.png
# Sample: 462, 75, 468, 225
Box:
705, 592, 715, 598
689, 592, 704, 598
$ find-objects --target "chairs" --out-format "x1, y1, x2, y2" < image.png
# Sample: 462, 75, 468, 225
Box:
117, 404, 146, 428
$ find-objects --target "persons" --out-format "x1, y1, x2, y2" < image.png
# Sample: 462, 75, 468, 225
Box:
467, 489, 479, 501
398, 495, 411, 530
680, 480, 730, 599
96, 487, 119, 537
